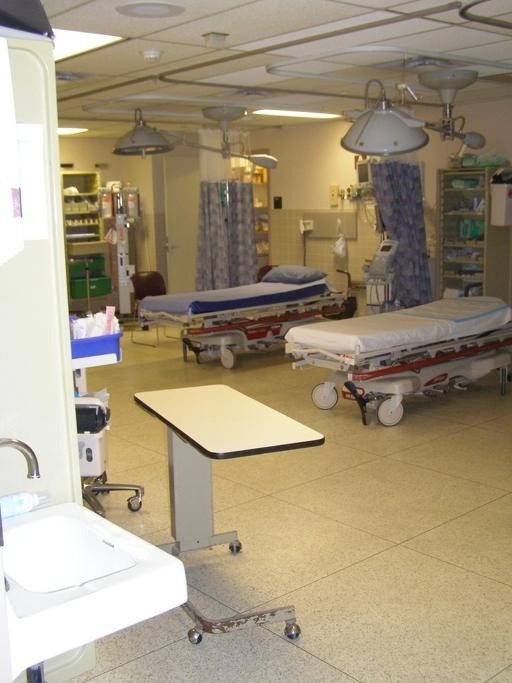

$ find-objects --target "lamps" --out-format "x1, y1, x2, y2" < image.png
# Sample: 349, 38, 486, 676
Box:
337, 62, 485, 160
110, 101, 277, 170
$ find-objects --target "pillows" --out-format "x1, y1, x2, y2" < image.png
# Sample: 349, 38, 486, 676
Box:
261, 265, 326, 284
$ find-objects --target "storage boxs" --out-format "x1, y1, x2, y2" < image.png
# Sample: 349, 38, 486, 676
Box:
68, 257, 112, 300
70, 318, 124, 363
77, 423, 111, 479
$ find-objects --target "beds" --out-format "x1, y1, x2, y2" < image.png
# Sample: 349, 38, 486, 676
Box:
140, 279, 347, 371
287, 296, 512, 430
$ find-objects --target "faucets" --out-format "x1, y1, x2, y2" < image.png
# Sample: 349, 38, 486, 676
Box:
0, 437, 40, 592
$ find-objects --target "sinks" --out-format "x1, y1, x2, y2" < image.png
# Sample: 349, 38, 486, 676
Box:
0, 490, 189, 683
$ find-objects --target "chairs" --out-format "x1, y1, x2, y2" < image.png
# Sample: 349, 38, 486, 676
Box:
131, 271, 185, 348
74, 394, 143, 518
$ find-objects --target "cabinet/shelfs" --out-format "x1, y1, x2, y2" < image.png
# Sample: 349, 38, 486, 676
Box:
231, 149, 270, 259
435, 166, 511, 305
62, 172, 114, 313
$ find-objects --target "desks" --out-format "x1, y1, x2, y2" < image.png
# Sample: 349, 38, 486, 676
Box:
133, 379, 324, 644
